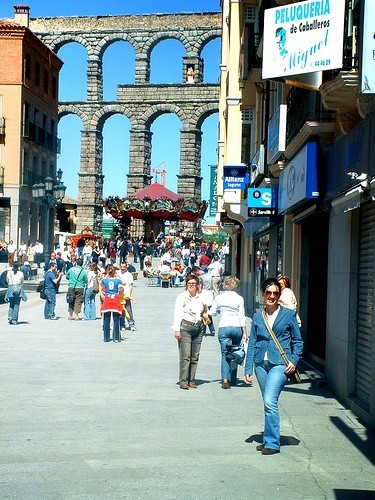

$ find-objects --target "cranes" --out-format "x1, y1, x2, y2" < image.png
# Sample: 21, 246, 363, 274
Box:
150, 161, 167, 185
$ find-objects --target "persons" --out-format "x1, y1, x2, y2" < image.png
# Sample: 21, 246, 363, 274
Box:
133, 229, 230, 294
244, 276, 304, 454
192, 266, 215, 335
208, 275, 248, 389
6, 261, 24, 324
0, 239, 44, 280
169, 226, 176, 234
255, 254, 282, 278
44, 235, 136, 343
174, 275, 209, 389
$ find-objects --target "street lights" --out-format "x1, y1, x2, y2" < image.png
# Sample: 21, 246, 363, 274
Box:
31, 175, 66, 263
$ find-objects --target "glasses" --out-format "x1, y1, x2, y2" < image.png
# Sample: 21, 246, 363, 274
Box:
265, 291, 280, 298
186, 283, 197, 286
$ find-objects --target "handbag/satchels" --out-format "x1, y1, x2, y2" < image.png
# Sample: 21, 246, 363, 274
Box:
93, 277, 99, 294
66, 292, 75, 302
291, 369, 301, 382
40, 281, 46, 299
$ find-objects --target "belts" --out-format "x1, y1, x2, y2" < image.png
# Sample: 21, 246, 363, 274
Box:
212, 276, 220, 277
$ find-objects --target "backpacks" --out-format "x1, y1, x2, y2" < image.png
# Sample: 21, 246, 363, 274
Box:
10, 271, 22, 293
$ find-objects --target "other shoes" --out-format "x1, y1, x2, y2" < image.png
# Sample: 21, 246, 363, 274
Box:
50, 317, 60, 320
190, 384, 197, 388
256, 444, 281, 454
122, 328, 125, 330
131, 326, 135, 331
180, 384, 189, 389
223, 380, 230, 388
